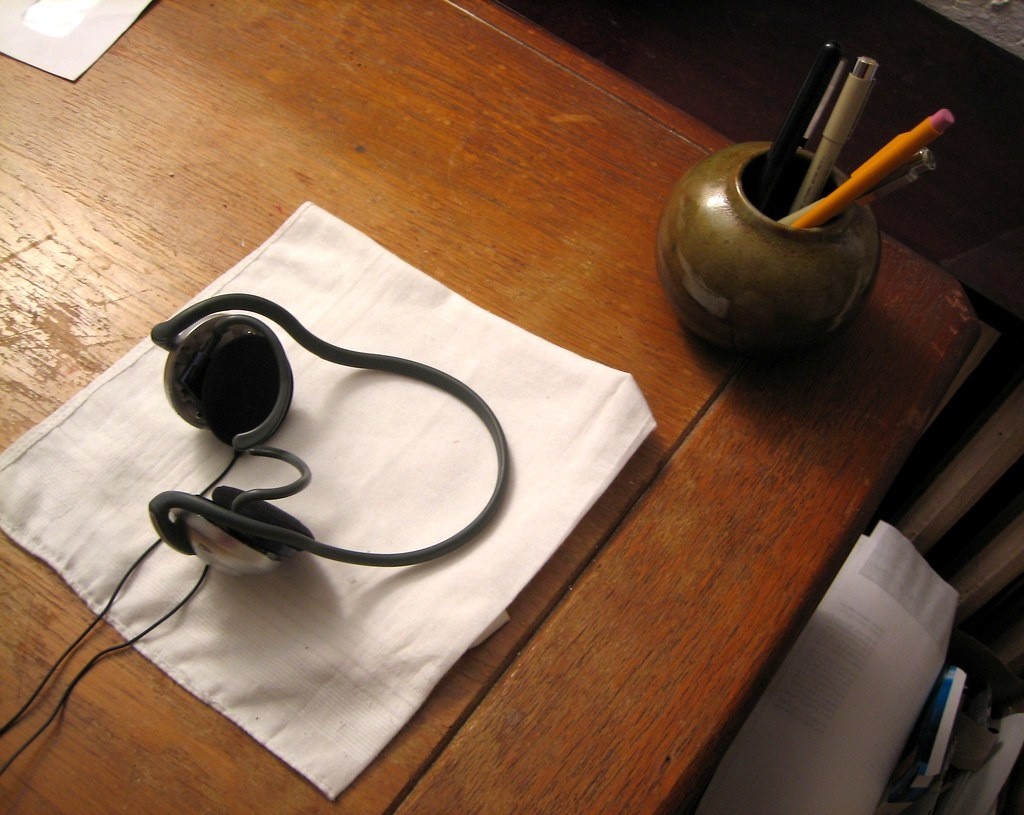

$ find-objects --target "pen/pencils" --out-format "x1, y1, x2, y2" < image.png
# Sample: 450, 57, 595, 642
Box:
751, 39, 956, 229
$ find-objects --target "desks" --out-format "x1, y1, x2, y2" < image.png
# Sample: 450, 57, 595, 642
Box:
0, 0, 976, 815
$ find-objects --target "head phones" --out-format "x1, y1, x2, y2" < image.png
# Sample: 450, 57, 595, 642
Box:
149, 293, 509, 580
